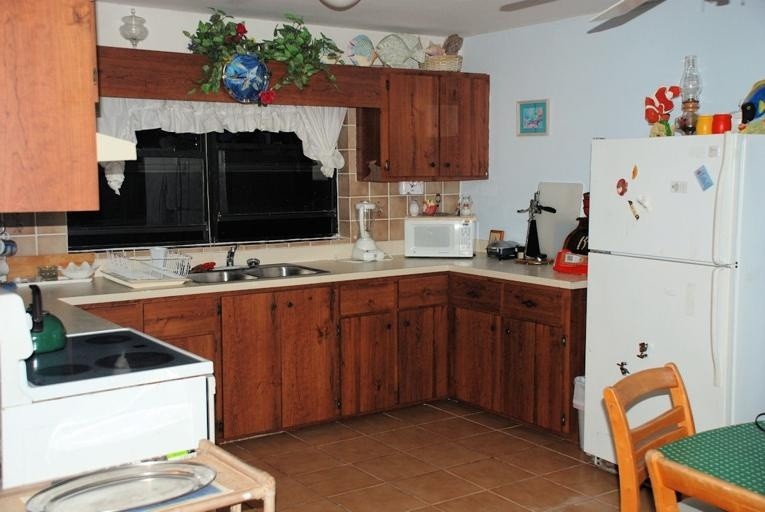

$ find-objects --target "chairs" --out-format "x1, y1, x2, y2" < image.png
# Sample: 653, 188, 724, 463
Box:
603, 359, 721, 509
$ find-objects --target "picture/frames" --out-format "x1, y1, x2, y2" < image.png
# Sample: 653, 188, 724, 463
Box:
515, 96, 552, 138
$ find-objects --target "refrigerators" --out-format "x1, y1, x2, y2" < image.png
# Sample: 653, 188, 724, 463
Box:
584, 131, 764, 491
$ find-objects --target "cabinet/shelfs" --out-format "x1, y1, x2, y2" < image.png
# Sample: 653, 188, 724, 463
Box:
451, 278, 566, 435
354, 73, 490, 183
141, 300, 215, 364
217, 286, 337, 440
337, 274, 452, 414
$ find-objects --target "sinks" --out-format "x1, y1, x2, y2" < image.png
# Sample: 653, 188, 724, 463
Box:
187, 268, 261, 286
245, 263, 331, 280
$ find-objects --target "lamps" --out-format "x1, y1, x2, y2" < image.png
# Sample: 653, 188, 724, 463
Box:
120, 6, 149, 47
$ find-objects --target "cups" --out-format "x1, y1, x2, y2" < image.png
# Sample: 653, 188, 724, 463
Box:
151, 247, 165, 267
0, 232, 18, 257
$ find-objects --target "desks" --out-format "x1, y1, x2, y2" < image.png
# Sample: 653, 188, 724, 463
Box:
643, 417, 765, 512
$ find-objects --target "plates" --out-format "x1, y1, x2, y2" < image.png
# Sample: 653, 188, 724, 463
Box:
25, 461, 217, 511
223, 54, 270, 102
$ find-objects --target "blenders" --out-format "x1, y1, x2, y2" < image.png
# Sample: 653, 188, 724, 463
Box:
351, 201, 380, 259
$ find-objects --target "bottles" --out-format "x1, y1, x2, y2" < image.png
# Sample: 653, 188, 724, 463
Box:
696, 113, 734, 134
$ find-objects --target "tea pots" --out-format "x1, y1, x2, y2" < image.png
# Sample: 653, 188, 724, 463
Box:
18, 284, 68, 353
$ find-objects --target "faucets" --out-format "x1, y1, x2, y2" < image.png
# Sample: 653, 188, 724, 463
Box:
226, 243, 237, 265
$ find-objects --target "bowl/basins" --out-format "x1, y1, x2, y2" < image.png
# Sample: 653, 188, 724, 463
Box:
58, 258, 99, 280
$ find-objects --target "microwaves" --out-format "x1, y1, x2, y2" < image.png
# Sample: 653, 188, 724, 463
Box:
401, 215, 475, 259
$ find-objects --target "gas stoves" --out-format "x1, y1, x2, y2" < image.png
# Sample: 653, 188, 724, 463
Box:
0, 288, 200, 407
